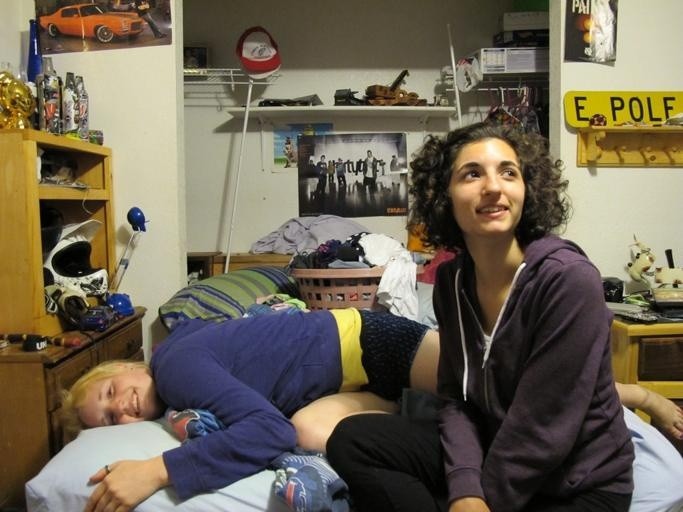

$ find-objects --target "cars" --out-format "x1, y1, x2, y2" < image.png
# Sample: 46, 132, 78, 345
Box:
39, 3, 146, 43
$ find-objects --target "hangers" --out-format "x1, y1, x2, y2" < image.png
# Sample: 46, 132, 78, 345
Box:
483, 82, 548, 114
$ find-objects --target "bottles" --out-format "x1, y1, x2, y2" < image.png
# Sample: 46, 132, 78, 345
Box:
26, 17, 90, 143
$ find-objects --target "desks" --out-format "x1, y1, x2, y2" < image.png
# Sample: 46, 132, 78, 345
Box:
607, 307, 681, 424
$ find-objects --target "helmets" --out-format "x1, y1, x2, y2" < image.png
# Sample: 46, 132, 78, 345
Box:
43, 220, 108, 297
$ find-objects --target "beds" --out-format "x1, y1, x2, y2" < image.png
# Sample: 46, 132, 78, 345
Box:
21, 415, 683, 509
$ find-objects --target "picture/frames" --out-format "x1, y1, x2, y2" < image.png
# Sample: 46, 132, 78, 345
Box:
183, 42, 211, 77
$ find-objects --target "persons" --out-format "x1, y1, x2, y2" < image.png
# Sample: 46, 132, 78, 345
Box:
362, 150, 378, 193
315, 155, 345, 195
325, 119, 637, 512
135, 0, 168, 38
58, 306, 683, 512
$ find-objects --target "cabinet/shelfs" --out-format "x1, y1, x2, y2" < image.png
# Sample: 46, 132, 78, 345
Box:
3, 131, 148, 507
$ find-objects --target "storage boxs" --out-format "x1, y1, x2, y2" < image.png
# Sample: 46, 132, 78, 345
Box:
466, 11, 548, 78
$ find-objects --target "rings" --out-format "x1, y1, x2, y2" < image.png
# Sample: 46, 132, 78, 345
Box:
105, 465, 110, 473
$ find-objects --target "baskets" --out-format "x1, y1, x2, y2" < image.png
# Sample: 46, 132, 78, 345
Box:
292, 268, 384, 311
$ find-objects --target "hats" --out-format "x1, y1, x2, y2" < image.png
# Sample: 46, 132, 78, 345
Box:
236, 26, 281, 73
